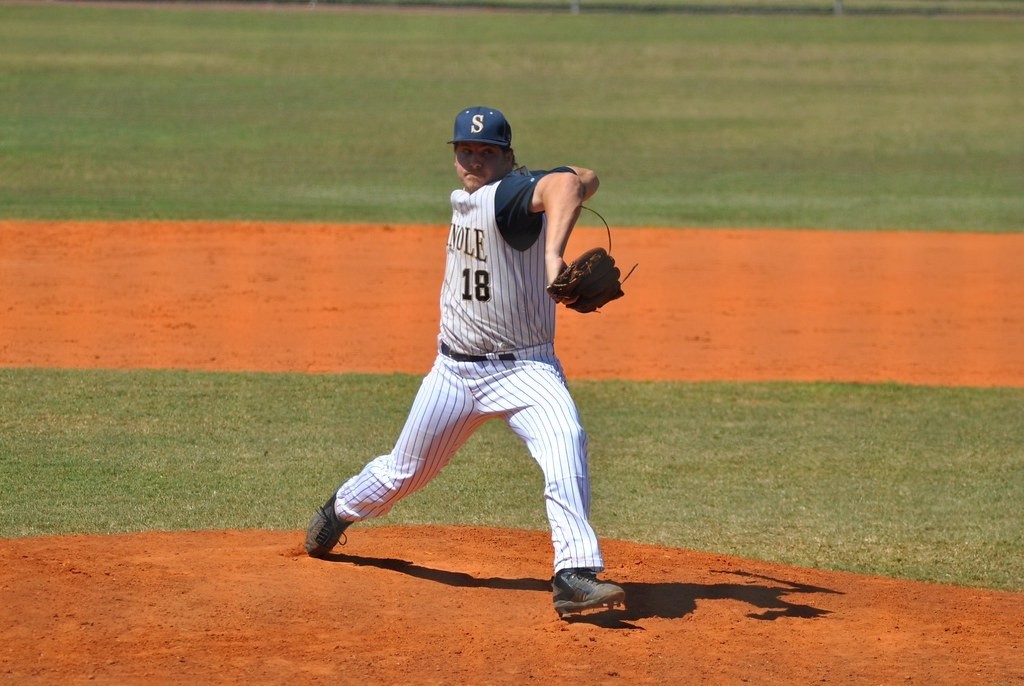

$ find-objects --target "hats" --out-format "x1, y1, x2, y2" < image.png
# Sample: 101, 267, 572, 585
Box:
446, 106, 513, 148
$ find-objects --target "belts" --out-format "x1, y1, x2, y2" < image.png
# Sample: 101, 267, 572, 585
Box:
438, 341, 517, 362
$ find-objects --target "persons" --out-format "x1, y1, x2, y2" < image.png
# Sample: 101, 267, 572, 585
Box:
299, 104, 626, 618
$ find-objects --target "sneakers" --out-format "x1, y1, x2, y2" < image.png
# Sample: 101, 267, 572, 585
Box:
304, 490, 354, 557
549, 567, 625, 619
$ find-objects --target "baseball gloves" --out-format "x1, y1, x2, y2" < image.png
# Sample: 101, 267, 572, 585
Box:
553, 246, 625, 313
